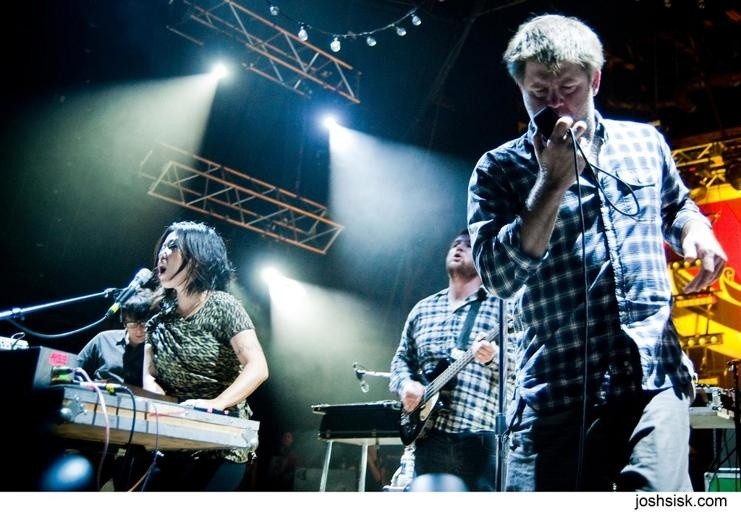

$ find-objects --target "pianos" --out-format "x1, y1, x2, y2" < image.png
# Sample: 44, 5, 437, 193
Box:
2, 383, 263, 452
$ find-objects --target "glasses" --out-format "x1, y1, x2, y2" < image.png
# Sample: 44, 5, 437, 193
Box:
124, 320, 148, 329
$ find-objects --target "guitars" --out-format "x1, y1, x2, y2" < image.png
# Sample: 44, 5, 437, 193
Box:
399, 313, 515, 446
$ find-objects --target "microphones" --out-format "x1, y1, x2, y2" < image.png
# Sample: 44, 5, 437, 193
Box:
532, 104, 598, 188
351, 361, 370, 393
106, 266, 155, 328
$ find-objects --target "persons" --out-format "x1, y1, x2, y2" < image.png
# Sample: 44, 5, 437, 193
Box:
70, 288, 154, 491
390, 227, 517, 492
138, 220, 270, 491
281, 430, 295, 444
355, 445, 397, 491
465, 12, 731, 492
